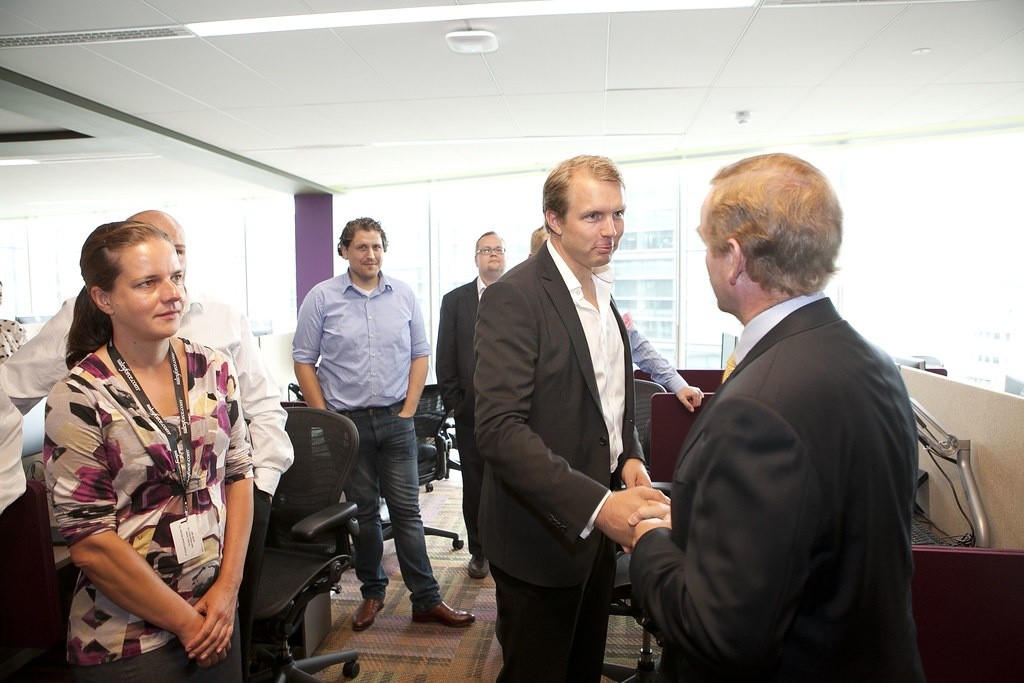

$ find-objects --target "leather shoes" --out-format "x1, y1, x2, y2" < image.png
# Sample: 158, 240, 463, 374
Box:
352, 599, 384, 631
412, 601, 475, 627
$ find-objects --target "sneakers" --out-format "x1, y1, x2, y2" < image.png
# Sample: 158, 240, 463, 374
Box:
468, 554, 489, 579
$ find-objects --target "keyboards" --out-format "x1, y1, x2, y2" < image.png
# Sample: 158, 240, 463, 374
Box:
911, 517, 954, 546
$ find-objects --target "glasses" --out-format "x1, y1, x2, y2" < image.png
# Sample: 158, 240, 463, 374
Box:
475, 247, 506, 255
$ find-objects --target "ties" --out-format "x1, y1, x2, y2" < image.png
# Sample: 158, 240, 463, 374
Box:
723, 348, 737, 382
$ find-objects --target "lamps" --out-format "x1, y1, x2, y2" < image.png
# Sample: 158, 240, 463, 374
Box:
445, 30, 499, 55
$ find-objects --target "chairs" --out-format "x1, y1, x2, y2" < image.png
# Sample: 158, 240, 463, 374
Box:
0, 482, 71, 647
382, 383, 464, 548
602, 482, 673, 683
249, 406, 358, 683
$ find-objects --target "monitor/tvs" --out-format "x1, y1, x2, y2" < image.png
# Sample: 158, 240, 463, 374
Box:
893, 355, 926, 370
721, 334, 739, 370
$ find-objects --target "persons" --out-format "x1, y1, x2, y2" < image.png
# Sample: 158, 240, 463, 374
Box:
292, 218, 475, 630
436, 231, 511, 579
0, 211, 294, 683
473, 155, 655, 683
528, 225, 704, 412
622, 152, 919, 683
